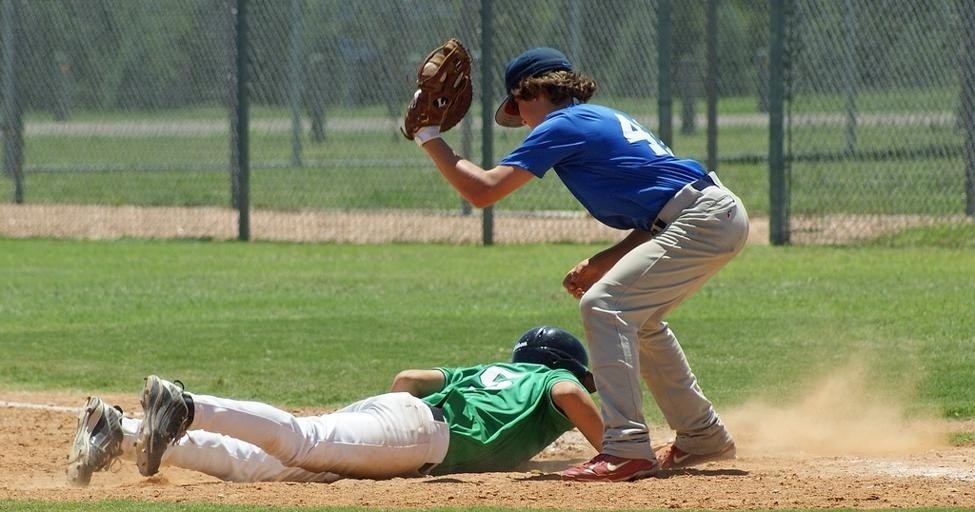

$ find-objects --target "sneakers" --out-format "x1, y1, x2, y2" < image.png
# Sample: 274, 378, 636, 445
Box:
560, 441, 736, 483
64, 396, 125, 486
133, 375, 189, 477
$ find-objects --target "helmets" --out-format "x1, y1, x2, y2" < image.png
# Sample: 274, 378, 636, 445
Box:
512, 326, 596, 393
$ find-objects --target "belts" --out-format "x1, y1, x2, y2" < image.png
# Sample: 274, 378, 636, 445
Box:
650, 177, 715, 237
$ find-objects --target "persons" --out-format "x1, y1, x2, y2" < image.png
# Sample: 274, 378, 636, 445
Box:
398, 36, 751, 483
62, 324, 606, 491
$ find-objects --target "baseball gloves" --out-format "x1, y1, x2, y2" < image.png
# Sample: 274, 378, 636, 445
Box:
399, 39, 472, 140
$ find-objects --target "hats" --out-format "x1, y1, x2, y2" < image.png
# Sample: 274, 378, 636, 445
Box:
495, 47, 572, 127
